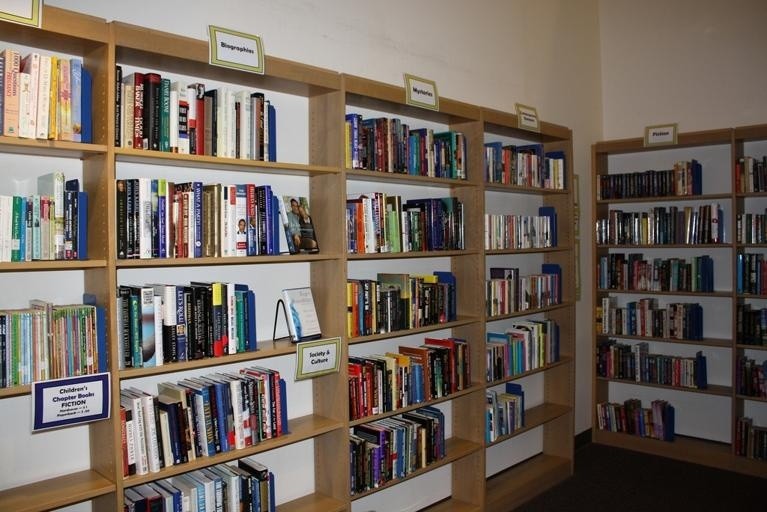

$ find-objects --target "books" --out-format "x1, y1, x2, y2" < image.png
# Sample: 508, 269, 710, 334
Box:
346, 337, 471, 421
343, 191, 465, 254
114, 66, 277, 163
345, 271, 457, 337
282, 287, 322, 343
484, 266, 559, 382
0, 49, 92, 143
344, 114, 466, 180
596, 398, 675, 442
1, 300, 109, 389
485, 389, 522, 444
596, 160, 724, 390
349, 405, 447, 497
115, 282, 257, 368
116, 178, 320, 260
119, 365, 289, 478
0, 172, 88, 262
124, 457, 275, 512
736, 157, 767, 456
485, 213, 552, 252
484, 144, 563, 190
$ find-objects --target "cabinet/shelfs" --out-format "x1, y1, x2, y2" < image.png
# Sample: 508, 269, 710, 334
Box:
343, 74, 485, 511
591, 128, 731, 470
485, 105, 575, 510
114, 20, 349, 511
0, 0, 120, 511
733, 124, 767, 483
